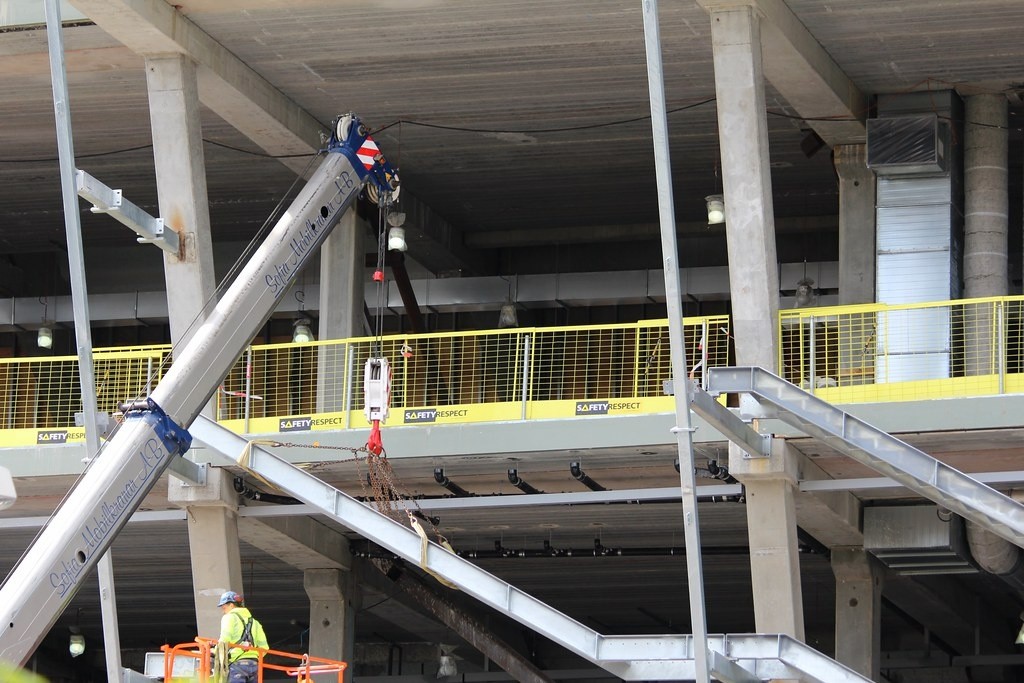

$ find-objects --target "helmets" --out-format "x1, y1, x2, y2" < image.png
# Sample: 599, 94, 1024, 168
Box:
217, 591, 243, 607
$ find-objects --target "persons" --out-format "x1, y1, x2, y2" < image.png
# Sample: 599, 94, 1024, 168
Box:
210, 590, 270, 683
